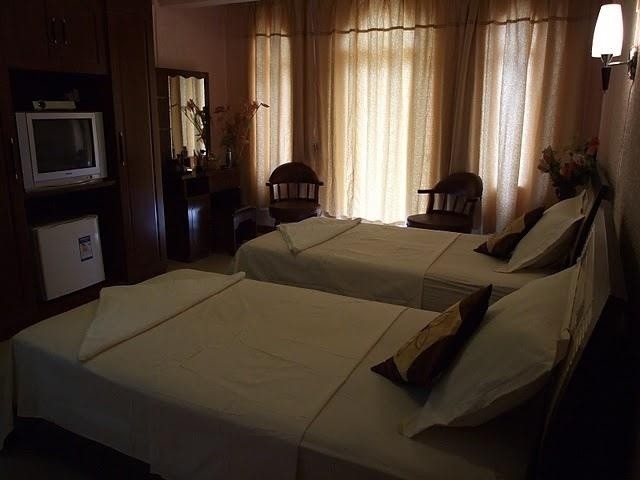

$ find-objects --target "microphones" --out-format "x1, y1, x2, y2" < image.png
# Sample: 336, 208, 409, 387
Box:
200, 204, 258, 257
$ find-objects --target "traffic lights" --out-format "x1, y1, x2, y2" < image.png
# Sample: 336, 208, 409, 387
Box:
370, 282, 493, 396
473, 204, 546, 261
397, 256, 583, 439
495, 189, 587, 273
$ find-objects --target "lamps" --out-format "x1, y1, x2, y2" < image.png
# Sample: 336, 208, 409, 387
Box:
266, 161, 324, 231
407, 172, 483, 234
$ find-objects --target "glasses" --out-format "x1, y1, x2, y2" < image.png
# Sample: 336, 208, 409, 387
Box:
591, 4, 638, 96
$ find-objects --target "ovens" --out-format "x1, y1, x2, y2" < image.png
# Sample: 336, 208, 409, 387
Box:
236, 165, 614, 312
10, 199, 640, 479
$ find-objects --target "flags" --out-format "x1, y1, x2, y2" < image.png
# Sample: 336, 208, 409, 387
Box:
226, 151, 233, 168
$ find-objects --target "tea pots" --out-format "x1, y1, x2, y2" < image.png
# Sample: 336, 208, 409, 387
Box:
156, 68, 213, 174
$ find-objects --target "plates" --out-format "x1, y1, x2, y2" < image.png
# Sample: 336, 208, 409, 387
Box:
163, 167, 240, 263
1, 0, 165, 339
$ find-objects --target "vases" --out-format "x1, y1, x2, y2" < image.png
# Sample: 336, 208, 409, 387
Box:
215, 100, 270, 157
170, 99, 208, 152
536, 134, 601, 200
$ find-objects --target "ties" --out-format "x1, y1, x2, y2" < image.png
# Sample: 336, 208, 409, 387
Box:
14, 111, 108, 191
